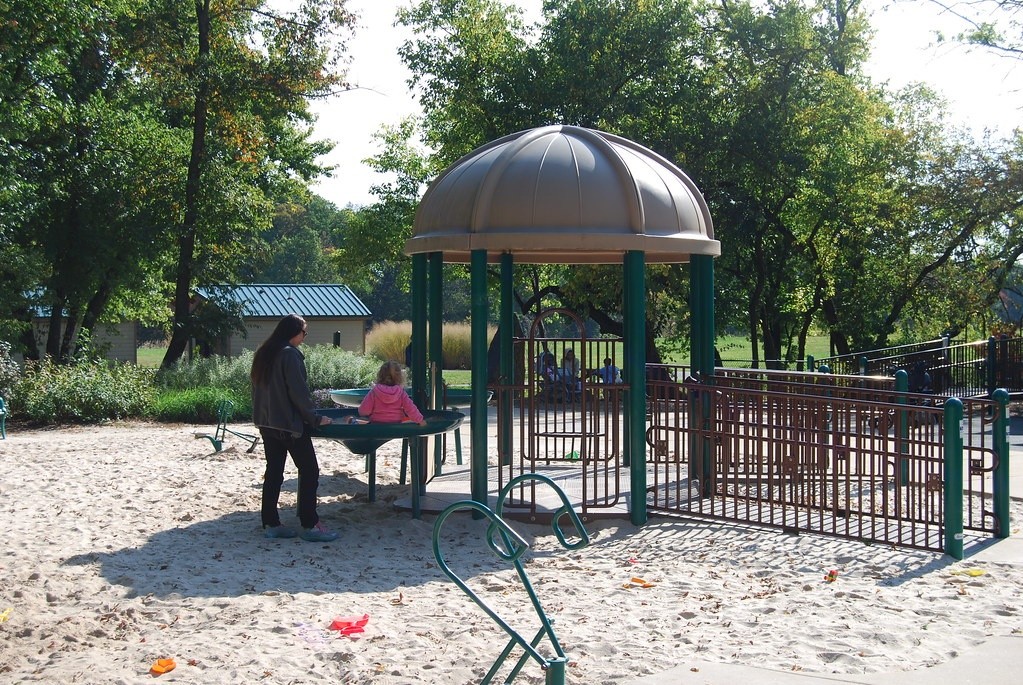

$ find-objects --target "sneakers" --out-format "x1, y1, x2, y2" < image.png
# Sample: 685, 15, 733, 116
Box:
263, 522, 297, 538
298, 521, 337, 542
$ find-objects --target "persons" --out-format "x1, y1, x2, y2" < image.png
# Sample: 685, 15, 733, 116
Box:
594, 357, 621, 400
561, 348, 579, 390
250, 313, 338, 541
348, 361, 428, 426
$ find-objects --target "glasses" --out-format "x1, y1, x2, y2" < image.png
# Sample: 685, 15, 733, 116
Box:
302, 330, 308, 340
